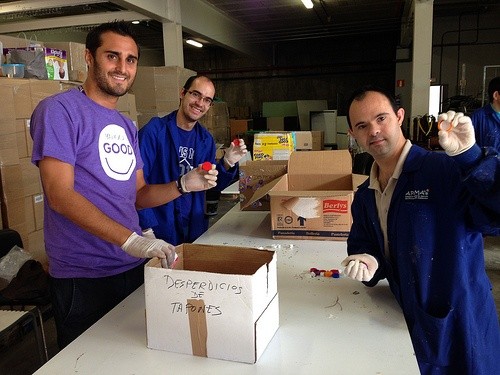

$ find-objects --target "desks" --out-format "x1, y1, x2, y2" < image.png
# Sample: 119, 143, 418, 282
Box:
221, 179, 240, 205
31, 202, 421, 375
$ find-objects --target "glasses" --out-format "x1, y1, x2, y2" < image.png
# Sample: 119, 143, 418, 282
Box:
186, 89, 214, 107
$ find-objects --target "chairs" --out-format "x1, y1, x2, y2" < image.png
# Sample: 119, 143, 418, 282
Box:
0, 229, 55, 362
0, 310, 43, 366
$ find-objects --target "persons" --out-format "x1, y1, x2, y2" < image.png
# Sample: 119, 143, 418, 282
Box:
472, 78, 500, 235
342, 87, 500, 375
136, 75, 247, 247
30, 19, 218, 350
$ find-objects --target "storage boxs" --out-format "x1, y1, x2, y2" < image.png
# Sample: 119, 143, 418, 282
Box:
0, 35, 369, 365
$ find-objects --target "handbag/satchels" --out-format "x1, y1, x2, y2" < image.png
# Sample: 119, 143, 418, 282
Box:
9, 31, 47, 80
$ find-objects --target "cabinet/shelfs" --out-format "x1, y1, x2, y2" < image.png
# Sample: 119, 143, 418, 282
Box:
230, 118, 254, 136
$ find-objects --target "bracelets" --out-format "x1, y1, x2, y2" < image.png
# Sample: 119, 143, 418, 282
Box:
177, 175, 185, 195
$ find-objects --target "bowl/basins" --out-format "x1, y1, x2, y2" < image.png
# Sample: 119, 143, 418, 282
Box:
1, 63, 25, 78
